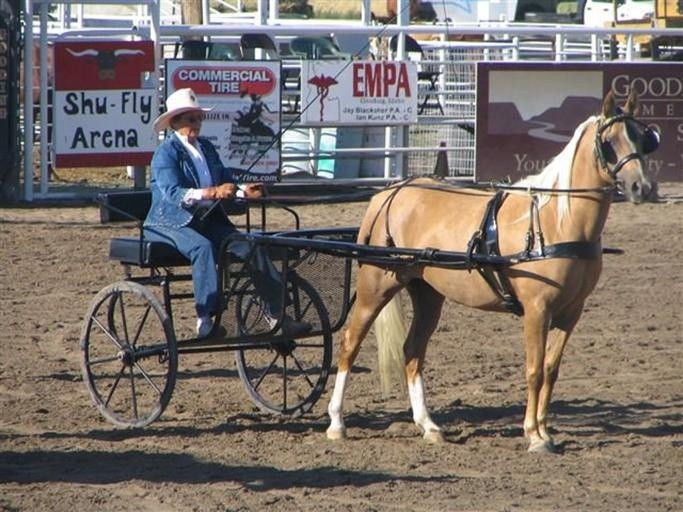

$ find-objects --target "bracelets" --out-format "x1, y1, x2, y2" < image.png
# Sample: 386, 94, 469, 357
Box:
208, 186, 216, 200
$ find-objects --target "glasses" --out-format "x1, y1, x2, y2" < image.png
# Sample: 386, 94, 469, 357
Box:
183, 114, 203, 125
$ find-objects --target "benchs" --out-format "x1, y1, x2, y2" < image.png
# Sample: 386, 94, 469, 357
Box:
97, 184, 303, 269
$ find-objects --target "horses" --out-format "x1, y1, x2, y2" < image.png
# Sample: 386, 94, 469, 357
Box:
325, 89, 654, 456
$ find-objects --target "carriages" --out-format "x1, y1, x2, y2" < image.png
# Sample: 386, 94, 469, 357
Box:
74, 84, 664, 459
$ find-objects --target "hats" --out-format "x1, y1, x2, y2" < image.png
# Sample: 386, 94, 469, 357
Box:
153, 87, 217, 133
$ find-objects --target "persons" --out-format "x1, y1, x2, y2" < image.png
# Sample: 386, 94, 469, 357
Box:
142, 86, 312, 336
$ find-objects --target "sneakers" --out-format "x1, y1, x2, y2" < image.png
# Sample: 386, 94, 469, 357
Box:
269, 310, 313, 337
196, 316, 226, 338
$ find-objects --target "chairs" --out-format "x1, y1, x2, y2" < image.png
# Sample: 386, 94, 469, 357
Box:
172, 33, 443, 115
